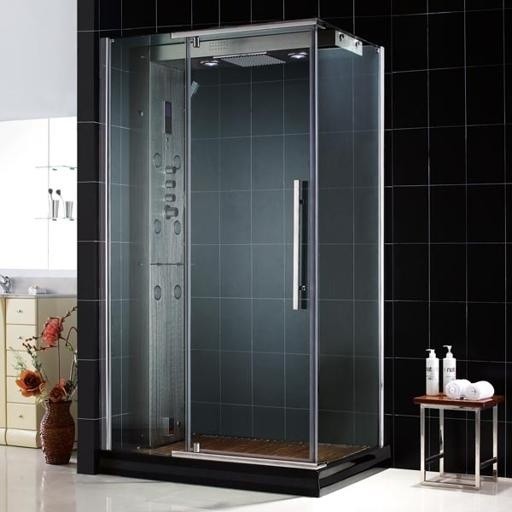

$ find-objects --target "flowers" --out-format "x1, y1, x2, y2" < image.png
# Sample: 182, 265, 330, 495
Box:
15, 307, 77, 401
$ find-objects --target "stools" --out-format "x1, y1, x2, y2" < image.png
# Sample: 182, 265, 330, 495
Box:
412, 394, 504, 491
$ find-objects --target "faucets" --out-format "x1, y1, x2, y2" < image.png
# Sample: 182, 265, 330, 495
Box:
0, 274, 10, 292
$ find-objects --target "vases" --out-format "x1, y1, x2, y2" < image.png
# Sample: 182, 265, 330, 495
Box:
39, 398, 76, 464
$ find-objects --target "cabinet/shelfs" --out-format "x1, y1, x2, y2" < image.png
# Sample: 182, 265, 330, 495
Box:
3, 297, 37, 431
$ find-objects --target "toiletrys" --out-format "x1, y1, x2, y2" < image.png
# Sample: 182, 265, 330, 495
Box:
443, 344, 456, 395
425, 349, 440, 396
